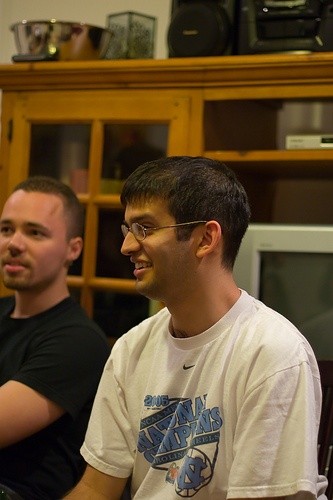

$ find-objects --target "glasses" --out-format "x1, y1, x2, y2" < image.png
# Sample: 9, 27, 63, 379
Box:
120, 220, 208, 241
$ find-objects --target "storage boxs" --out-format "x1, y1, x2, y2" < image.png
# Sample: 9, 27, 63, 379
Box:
106, 11, 157, 60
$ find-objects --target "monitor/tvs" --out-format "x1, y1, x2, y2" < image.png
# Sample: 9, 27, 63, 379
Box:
233, 224, 333, 388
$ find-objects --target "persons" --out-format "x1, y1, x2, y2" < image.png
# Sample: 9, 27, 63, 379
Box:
0, 177, 131, 500
61, 156, 322, 500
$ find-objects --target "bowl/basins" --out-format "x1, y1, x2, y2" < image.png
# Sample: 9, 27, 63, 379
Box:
11, 20, 114, 61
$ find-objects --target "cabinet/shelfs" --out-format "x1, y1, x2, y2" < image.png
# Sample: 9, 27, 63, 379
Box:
0, 52, 333, 346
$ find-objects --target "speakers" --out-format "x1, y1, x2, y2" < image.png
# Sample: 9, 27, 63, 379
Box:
166, 0, 239, 56
235, 0, 333, 53
